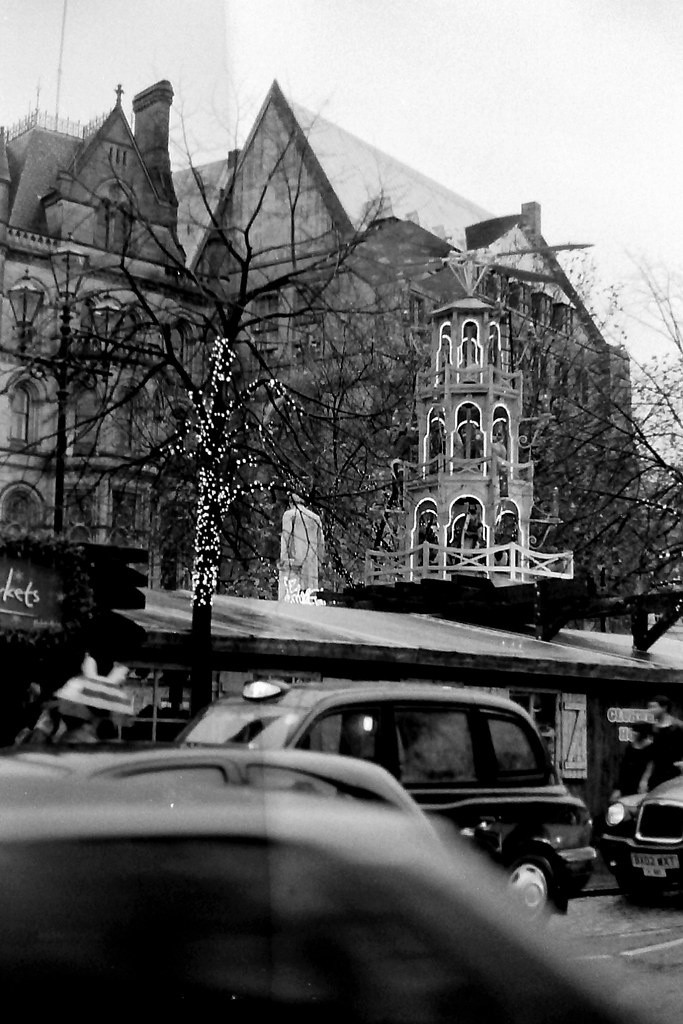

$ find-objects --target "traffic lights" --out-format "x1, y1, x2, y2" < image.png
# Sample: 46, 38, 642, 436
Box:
94, 544, 151, 663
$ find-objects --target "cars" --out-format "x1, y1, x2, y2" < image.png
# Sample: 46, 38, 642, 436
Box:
161, 682, 593, 919
0, 740, 447, 861
0, 777, 683, 1024
591, 772, 683, 908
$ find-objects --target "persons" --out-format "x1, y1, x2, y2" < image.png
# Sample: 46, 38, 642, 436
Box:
280, 494, 325, 591
647, 693, 683, 792
609, 722, 652, 802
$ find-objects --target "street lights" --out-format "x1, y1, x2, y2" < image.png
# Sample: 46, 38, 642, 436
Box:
7, 232, 126, 551
